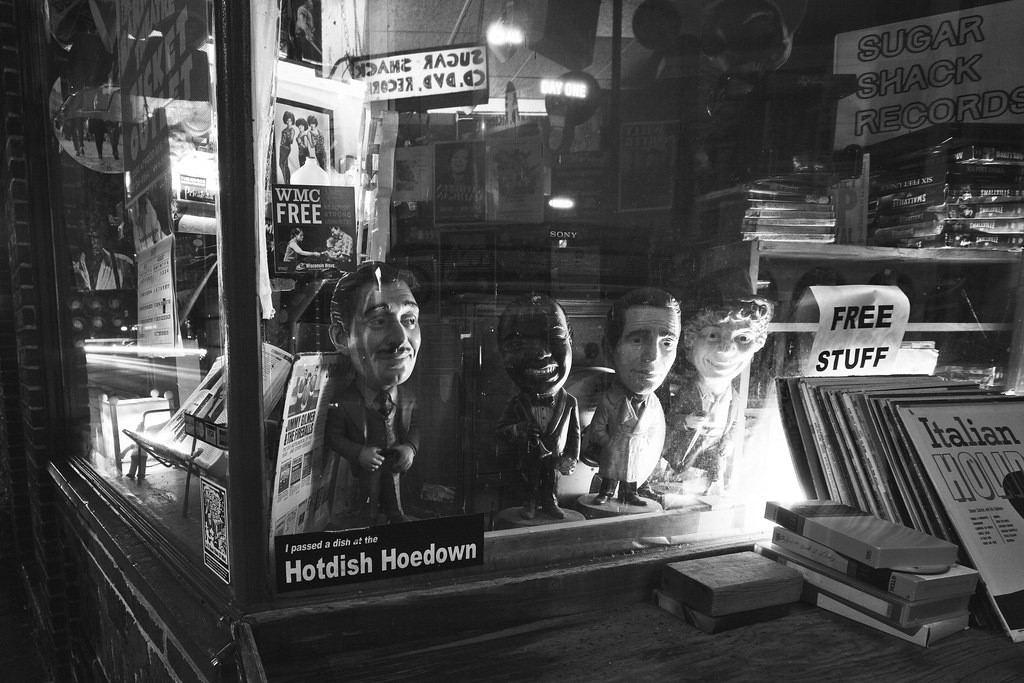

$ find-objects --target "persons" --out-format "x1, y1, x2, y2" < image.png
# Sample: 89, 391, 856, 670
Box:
67, 112, 120, 161
325, 261, 421, 527
497, 293, 581, 520
439, 148, 477, 212
581, 287, 682, 508
71, 218, 133, 290
654, 269, 775, 497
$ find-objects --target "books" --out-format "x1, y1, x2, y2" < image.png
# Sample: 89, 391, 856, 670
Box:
756, 376, 1024, 644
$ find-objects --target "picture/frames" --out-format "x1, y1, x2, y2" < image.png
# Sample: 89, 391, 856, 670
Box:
272, 97, 334, 185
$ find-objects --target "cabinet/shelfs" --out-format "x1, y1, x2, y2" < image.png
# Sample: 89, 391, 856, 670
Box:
690, 240, 1024, 420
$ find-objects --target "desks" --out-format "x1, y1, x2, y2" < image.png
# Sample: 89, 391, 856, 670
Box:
247, 593, 1023, 683
